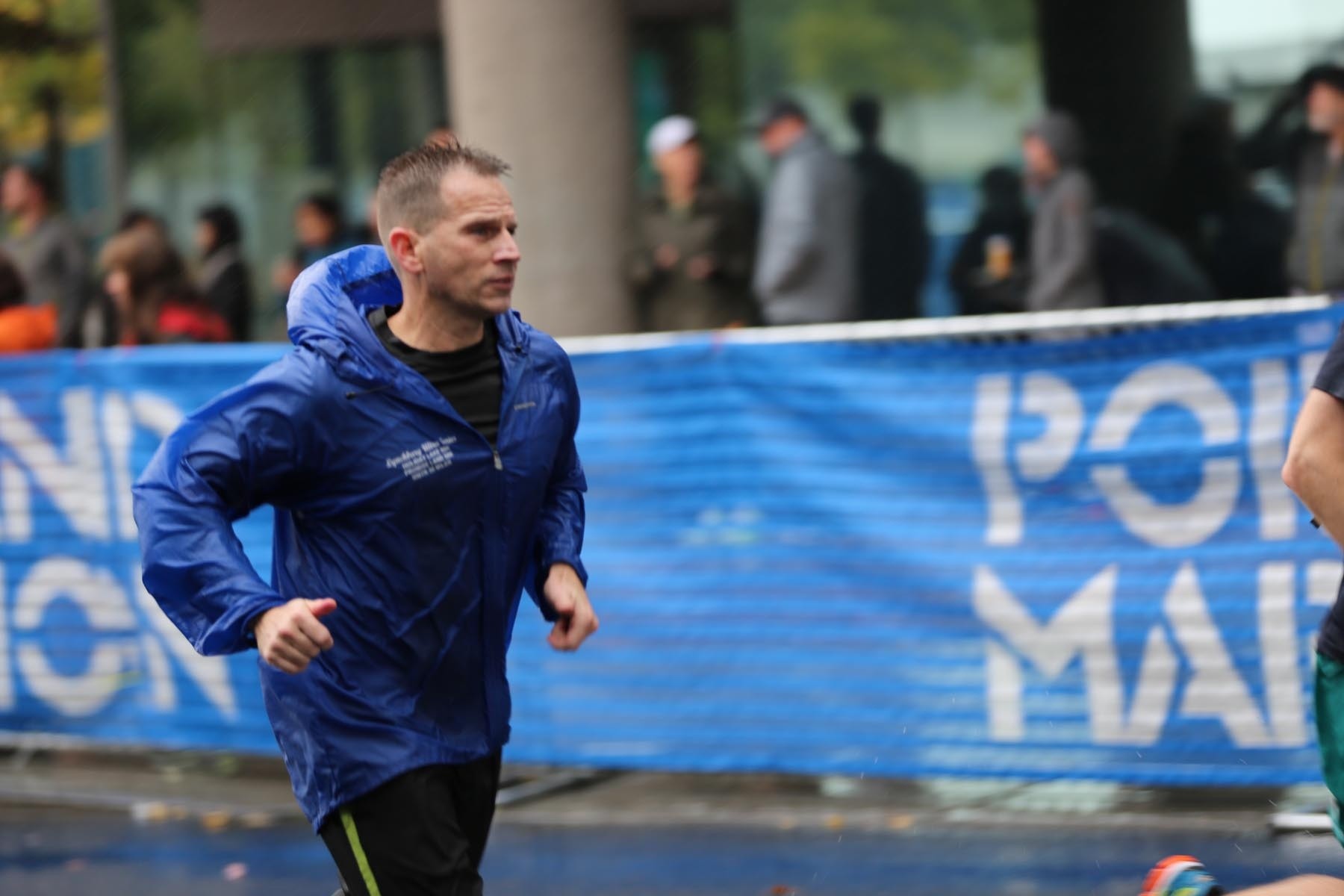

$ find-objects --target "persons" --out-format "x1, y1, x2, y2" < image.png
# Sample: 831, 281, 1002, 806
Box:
0, 164, 382, 351
130, 134, 601, 896
627, 60, 1344, 331
1139, 322, 1344, 896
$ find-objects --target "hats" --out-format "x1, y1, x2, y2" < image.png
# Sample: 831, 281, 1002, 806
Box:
647, 114, 697, 151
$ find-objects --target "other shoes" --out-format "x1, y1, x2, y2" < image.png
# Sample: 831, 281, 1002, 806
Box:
1135, 854, 1221, 896
